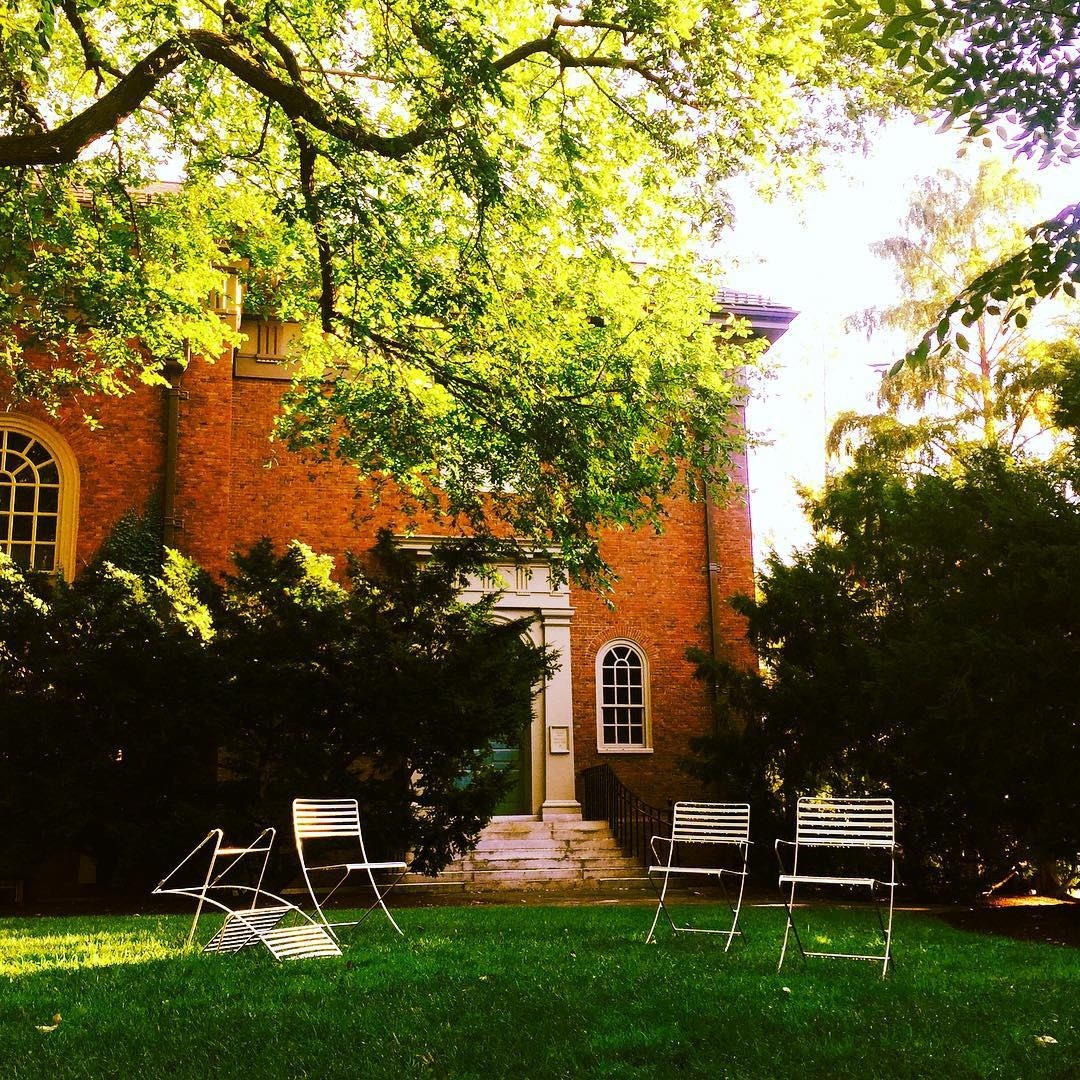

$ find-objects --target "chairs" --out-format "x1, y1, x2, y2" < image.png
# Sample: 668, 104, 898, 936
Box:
774, 796, 906, 982
645, 801, 756, 953
292, 797, 407, 948
152, 827, 344, 966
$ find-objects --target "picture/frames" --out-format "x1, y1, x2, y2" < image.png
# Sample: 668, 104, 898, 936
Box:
549, 725, 571, 755
